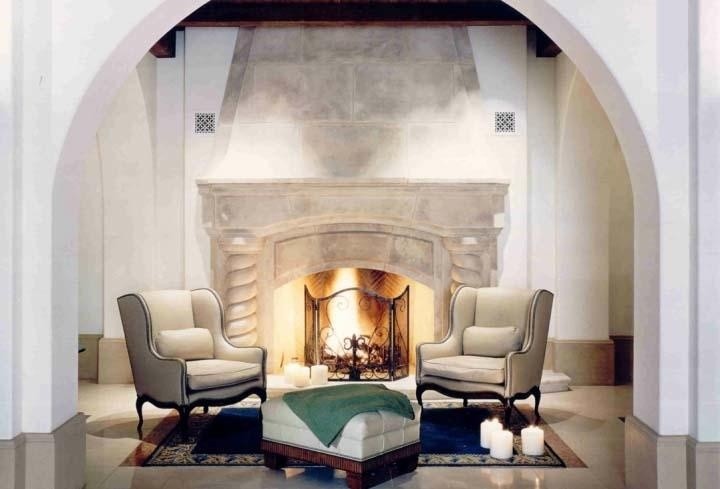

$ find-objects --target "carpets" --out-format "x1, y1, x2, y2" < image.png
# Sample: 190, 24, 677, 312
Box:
118, 399, 587, 468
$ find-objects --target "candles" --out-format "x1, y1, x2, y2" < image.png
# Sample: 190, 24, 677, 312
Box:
310, 364, 329, 384
283, 362, 303, 382
479, 417, 504, 449
293, 367, 310, 387
520, 424, 545, 457
490, 429, 513, 459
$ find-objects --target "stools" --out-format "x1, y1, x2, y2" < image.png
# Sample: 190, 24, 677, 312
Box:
260, 384, 422, 489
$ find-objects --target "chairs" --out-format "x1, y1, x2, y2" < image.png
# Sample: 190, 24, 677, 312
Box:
117, 286, 269, 443
415, 284, 554, 430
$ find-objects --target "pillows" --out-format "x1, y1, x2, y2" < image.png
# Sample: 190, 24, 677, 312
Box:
154, 327, 215, 360
461, 325, 522, 357
